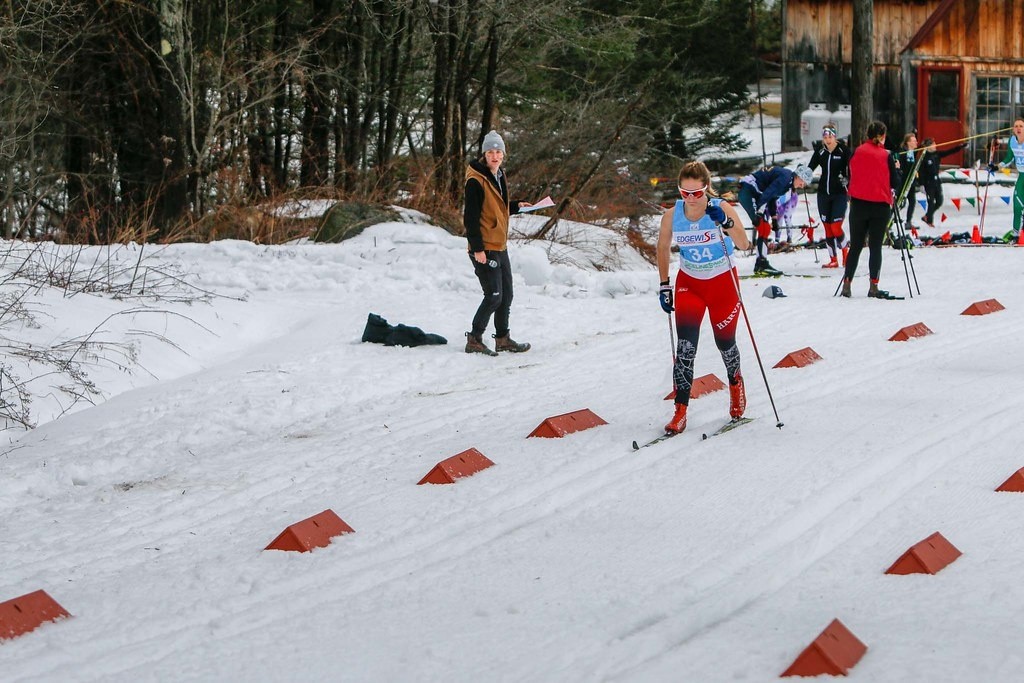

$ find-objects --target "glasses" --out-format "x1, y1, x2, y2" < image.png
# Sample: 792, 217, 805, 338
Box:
678, 185, 707, 199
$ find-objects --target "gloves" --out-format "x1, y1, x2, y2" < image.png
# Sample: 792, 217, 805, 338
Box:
919, 185, 926, 194
659, 281, 676, 314
987, 162, 998, 172
773, 219, 780, 231
753, 212, 764, 225
705, 205, 732, 229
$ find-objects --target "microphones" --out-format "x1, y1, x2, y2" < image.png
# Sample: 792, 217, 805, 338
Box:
469, 251, 497, 268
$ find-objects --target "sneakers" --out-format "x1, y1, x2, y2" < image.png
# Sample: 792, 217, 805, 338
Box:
492, 329, 531, 352
665, 404, 687, 433
465, 331, 499, 357
729, 376, 746, 418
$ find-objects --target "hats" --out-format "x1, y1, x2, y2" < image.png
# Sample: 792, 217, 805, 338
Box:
796, 166, 814, 184
482, 130, 505, 152
763, 286, 787, 300
822, 127, 837, 137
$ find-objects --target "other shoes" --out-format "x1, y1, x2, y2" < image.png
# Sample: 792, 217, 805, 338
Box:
754, 260, 783, 275
868, 287, 889, 297
905, 222, 920, 231
843, 284, 852, 298
922, 215, 935, 227
842, 255, 848, 267
822, 260, 839, 269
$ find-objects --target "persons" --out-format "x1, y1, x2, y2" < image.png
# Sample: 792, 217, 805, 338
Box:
797, 123, 851, 269
993, 135, 1000, 163
737, 164, 813, 275
917, 138, 971, 226
988, 118, 1024, 243
463, 129, 533, 357
892, 132, 920, 230
657, 163, 749, 434
839, 120, 901, 299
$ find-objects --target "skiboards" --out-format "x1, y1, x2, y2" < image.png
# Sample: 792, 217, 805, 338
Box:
882, 148, 926, 244
738, 273, 832, 280
838, 294, 905, 300
631, 415, 756, 450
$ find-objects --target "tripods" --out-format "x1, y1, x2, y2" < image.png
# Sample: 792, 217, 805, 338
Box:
833, 193, 921, 300
